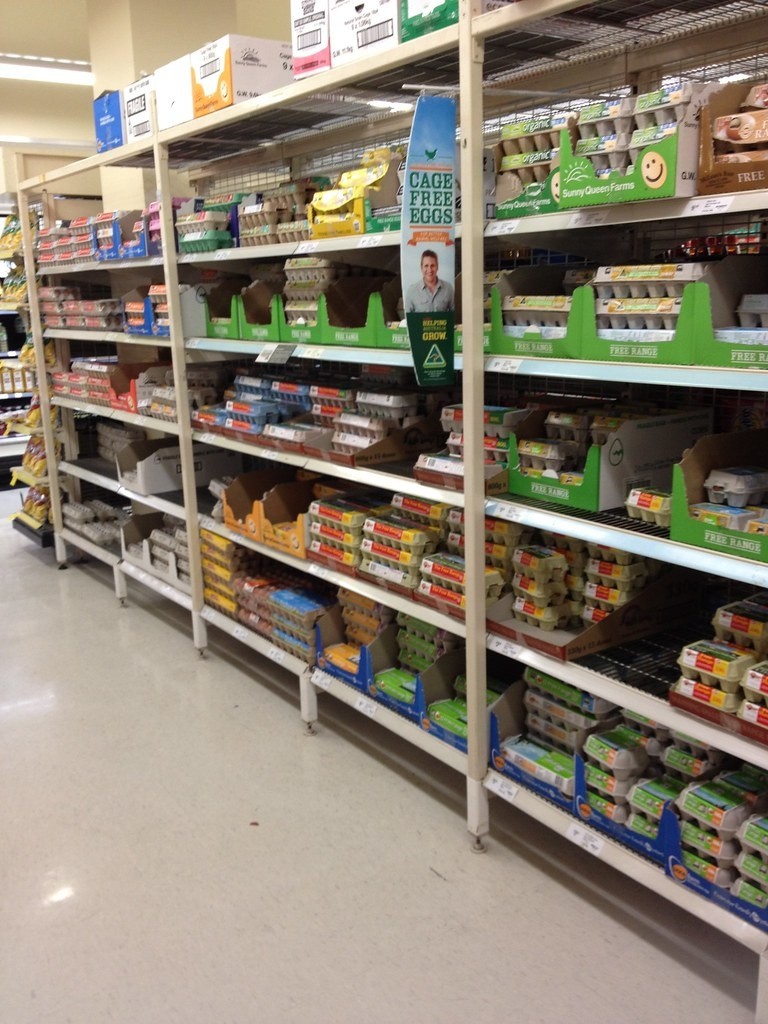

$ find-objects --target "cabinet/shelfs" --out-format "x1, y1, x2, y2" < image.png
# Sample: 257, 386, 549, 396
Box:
0, 0, 768, 1024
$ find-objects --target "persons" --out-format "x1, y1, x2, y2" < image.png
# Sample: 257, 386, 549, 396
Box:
405, 250, 454, 313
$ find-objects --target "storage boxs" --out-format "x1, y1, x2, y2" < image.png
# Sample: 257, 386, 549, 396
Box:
401, 0, 459, 44
192, 33, 291, 118
123, 74, 156, 143
669, 427, 768, 563
290, 0, 330, 81
155, 53, 193, 134
114, 436, 243, 495
92, 90, 126, 152
329, 0, 401, 68
507, 404, 713, 515
